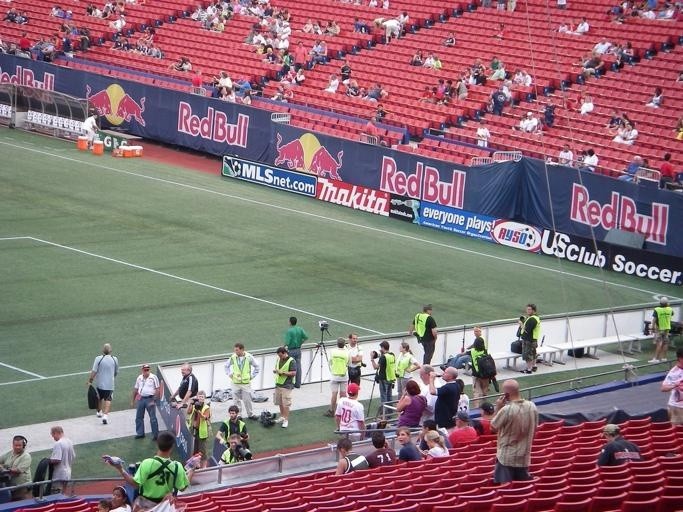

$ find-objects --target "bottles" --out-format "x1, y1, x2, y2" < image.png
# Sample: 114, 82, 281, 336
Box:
185, 451, 203, 464
183, 456, 201, 470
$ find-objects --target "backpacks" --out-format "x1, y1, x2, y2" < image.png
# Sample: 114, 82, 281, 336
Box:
86, 385, 99, 409
476, 349, 498, 381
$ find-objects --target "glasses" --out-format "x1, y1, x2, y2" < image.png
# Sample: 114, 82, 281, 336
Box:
143, 369, 150, 371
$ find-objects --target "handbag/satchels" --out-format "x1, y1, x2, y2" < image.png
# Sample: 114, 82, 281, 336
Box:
134, 394, 142, 401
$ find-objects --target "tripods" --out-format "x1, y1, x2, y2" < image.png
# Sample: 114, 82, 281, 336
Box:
302, 328, 331, 393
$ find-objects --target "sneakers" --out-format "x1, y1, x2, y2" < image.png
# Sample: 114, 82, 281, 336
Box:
647, 357, 660, 364
248, 415, 259, 420
236, 415, 242, 421
322, 408, 335, 418
96, 410, 103, 418
134, 434, 146, 440
520, 369, 533, 375
273, 417, 285, 423
102, 414, 108, 425
281, 419, 289, 429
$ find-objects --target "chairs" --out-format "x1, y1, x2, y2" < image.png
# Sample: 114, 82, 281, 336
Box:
1, 1, 683, 178
15, 498, 101, 512
175, 416, 683, 512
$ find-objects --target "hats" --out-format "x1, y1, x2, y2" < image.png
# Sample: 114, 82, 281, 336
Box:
451, 411, 470, 422
474, 401, 495, 414
141, 364, 150, 369
423, 429, 440, 442
660, 297, 670, 304
599, 422, 621, 438
346, 382, 361, 394
337, 336, 347, 345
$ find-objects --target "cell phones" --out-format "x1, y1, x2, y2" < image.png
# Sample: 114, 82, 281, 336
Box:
501, 393, 510, 401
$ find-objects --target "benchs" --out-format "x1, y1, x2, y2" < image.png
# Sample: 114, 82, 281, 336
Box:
461, 326, 683, 380
